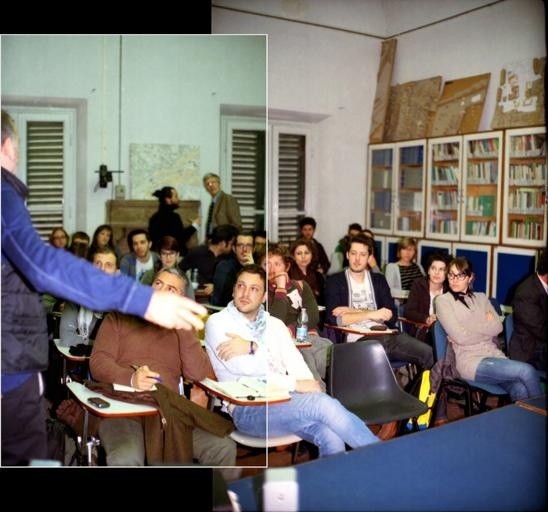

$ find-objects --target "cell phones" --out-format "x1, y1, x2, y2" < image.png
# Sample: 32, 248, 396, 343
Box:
88, 397, 110, 408
371, 326, 387, 331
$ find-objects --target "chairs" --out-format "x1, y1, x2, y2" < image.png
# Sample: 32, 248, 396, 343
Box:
324, 306, 414, 380
54, 339, 94, 384
506, 313, 548, 397
434, 318, 514, 409
67, 381, 158, 466
326, 341, 428, 429
398, 300, 434, 375
194, 377, 318, 459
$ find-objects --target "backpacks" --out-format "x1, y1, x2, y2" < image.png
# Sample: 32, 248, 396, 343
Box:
401, 369, 444, 434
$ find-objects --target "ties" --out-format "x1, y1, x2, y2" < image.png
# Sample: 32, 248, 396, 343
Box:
207, 202, 214, 233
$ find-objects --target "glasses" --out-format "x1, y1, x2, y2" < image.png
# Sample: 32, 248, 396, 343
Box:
447, 273, 465, 280
161, 251, 176, 257
54, 236, 65, 239
236, 243, 252, 247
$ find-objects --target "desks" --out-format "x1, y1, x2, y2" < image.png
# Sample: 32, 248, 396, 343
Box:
228, 405, 547, 509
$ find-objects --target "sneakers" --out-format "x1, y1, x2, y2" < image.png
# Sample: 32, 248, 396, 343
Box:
376, 421, 398, 441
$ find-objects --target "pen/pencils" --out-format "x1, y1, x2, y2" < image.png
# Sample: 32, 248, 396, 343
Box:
129, 364, 164, 383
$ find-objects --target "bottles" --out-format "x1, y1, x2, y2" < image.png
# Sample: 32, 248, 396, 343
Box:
191, 268, 200, 291
295, 308, 309, 344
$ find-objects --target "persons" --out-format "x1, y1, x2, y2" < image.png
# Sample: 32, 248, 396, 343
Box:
1, 109, 548, 484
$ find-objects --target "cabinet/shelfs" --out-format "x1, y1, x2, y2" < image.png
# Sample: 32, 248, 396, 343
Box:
427, 128, 504, 233
368, 235, 423, 275
418, 242, 491, 294
503, 126, 548, 249
494, 246, 535, 286
369, 138, 426, 239
